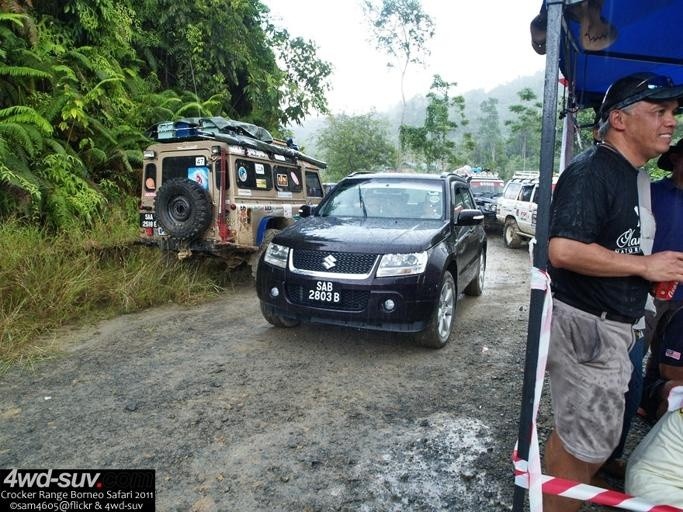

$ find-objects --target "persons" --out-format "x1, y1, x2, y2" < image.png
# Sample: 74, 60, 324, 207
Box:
539, 70, 681, 512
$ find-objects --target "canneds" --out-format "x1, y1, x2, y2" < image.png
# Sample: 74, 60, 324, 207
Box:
654, 281, 679, 301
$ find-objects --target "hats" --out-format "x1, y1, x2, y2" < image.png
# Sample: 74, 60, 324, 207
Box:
600, 71, 683, 123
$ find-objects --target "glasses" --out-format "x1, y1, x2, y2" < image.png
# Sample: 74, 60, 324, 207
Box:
620, 76, 672, 102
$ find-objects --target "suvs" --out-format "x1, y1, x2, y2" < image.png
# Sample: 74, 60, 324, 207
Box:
139, 139, 327, 284
255, 170, 488, 350
496, 170, 560, 249
465, 171, 505, 235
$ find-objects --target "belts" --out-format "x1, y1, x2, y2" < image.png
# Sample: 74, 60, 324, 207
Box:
552, 292, 637, 326
